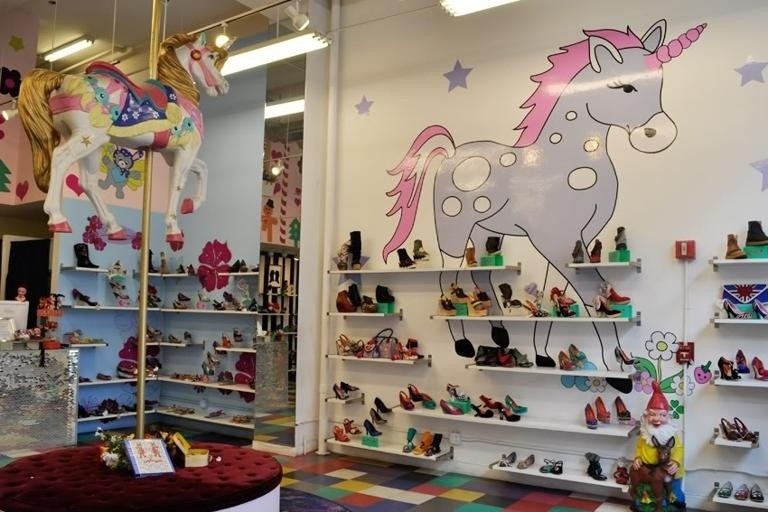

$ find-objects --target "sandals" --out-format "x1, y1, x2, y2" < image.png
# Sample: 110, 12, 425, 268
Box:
540, 459, 564, 474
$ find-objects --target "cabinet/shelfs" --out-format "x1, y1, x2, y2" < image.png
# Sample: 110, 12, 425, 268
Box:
324, 258, 767, 508
63, 260, 257, 441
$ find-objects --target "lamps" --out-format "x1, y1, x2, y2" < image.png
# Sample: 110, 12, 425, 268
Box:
263, 153, 302, 177
38, 33, 97, 67
203, 27, 336, 80
264, 94, 305, 120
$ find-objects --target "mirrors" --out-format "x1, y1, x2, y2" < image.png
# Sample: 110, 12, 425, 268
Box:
253, 13, 305, 448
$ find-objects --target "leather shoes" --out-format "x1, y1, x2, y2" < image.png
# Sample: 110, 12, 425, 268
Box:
77, 325, 252, 423
73, 244, 259, 310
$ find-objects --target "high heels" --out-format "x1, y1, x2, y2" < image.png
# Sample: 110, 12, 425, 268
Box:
474, 345, 534, 368
398, 382, 528, 421
614, 347, 635, 364
585, 396, 632, 429
438, 281, 632, 318
374, 398, 392, 413
334, 426, 349, 442
342, 419, 362, 434
369, 408, 387, 424
336, 335, 362, 356
333, 382, 360, 400
559, 344, 588, 371
364, 420, 383, 436
718, 220, 766, 502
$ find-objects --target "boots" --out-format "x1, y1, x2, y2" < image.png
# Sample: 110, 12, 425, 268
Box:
335, 283, 395, 312
464, 247, 478, 267
486, 236, 502, 256
336, 230, 364, 270
585, 452, 607, 480
590, 239, 602, 263
572, 240, 584, 263
615, 227, 627, 249
397, 240, 430, 269
403, 427, 442, 456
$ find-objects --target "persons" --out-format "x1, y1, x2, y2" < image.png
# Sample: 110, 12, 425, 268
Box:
14, 284, 29, 302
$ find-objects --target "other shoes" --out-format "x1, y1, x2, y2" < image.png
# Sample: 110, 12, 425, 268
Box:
613, 462, 629, 483
517, 455, 535, 468
500, 452, 516, 467
405, 338, 425, 359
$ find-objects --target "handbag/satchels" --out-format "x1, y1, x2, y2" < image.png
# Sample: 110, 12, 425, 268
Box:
363, 328, 404, 360
